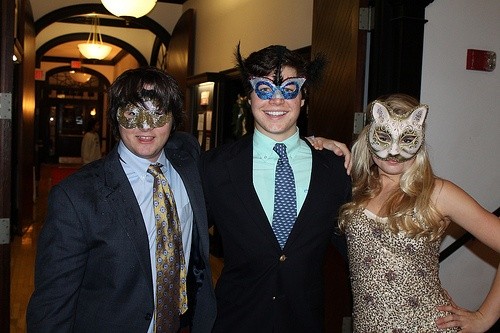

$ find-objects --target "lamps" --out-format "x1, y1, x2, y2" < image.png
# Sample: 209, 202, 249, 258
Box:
76, 15, 111, 60
101, 0, 157, 22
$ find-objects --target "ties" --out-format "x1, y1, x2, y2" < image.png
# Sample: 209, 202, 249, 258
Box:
147, 162, 189, 333
273, 143, 298, 249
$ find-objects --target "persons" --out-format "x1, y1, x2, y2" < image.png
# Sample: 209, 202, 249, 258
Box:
26, 67, 353, 333
80, 118, 102, 164
197, 44, 353, 333
304, 93, 500, 333
